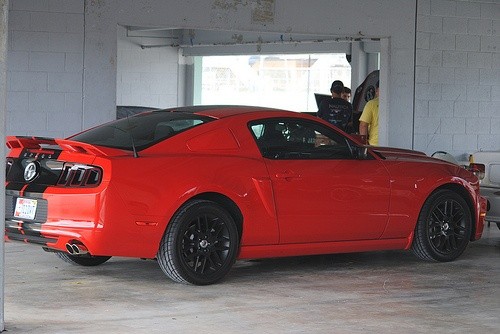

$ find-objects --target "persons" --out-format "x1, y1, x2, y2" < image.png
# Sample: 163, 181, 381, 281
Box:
341, 87, 351, 101
316, 80, 353, 144
359, 79, 379, 147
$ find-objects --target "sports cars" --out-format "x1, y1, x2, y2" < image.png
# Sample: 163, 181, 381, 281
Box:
3, 105, 500, 286
